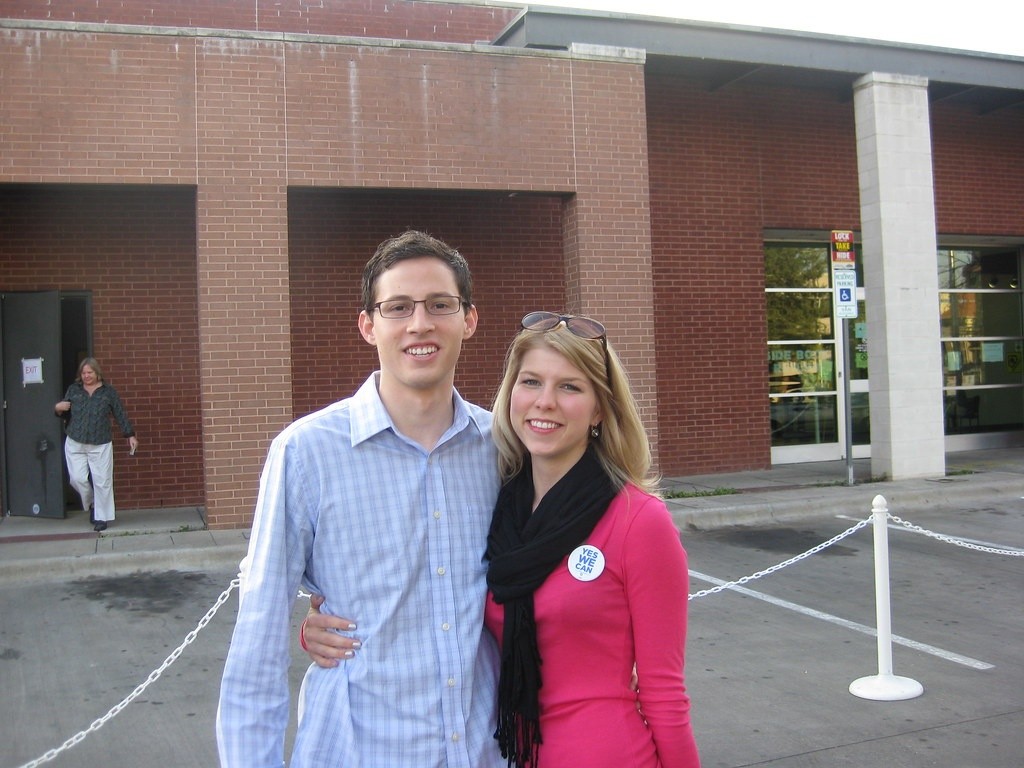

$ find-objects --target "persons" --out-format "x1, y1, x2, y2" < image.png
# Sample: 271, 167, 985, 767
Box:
301, 312, 703, 768
53, 358, 139, 532
214, 230, 642, 768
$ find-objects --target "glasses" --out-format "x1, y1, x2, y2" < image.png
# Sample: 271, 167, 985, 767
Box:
367, 296, 470, 319
519, 310, 608, 364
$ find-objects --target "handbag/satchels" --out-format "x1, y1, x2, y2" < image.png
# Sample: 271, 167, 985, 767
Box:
64, 399, 71, 429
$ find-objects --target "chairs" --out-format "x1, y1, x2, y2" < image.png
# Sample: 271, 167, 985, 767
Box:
948, 391, 980, 428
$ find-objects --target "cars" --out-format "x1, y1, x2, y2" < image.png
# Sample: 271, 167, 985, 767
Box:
771, 387, 870, 442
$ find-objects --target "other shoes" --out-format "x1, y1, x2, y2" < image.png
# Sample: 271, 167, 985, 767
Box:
94, 520, 107, 531
89, 506, 97, 524
